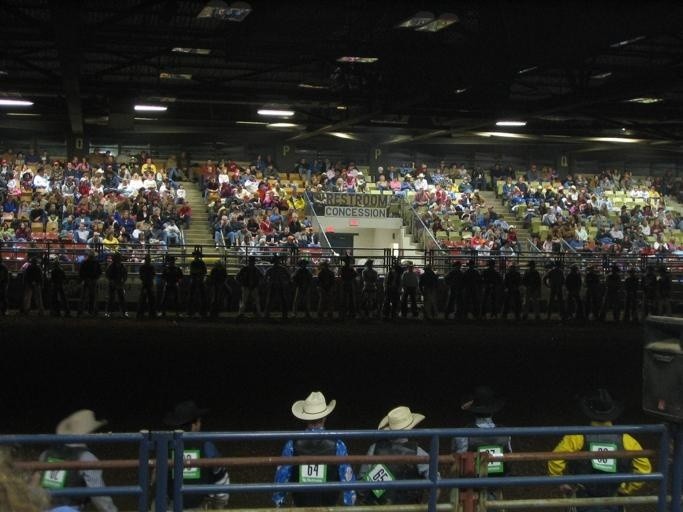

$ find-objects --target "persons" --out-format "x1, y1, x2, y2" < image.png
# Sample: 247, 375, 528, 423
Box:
29, 409, 119, 511
449, 383, 515, 512
0, 146, 683, 318
357, 406, 443, 504
549, 386, 653, 512
269, 388, 359, 507
157, 401, 231, 511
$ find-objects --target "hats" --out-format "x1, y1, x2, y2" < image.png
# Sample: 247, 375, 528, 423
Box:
163, 400, 210, 427
460, 391, 504, 414
291, 391, 337, 421
55, 409, 103, 435
377, 406, 426, 431
582, 387, 625, 421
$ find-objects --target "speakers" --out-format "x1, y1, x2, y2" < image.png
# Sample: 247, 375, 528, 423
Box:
640, 313, 683, 421
